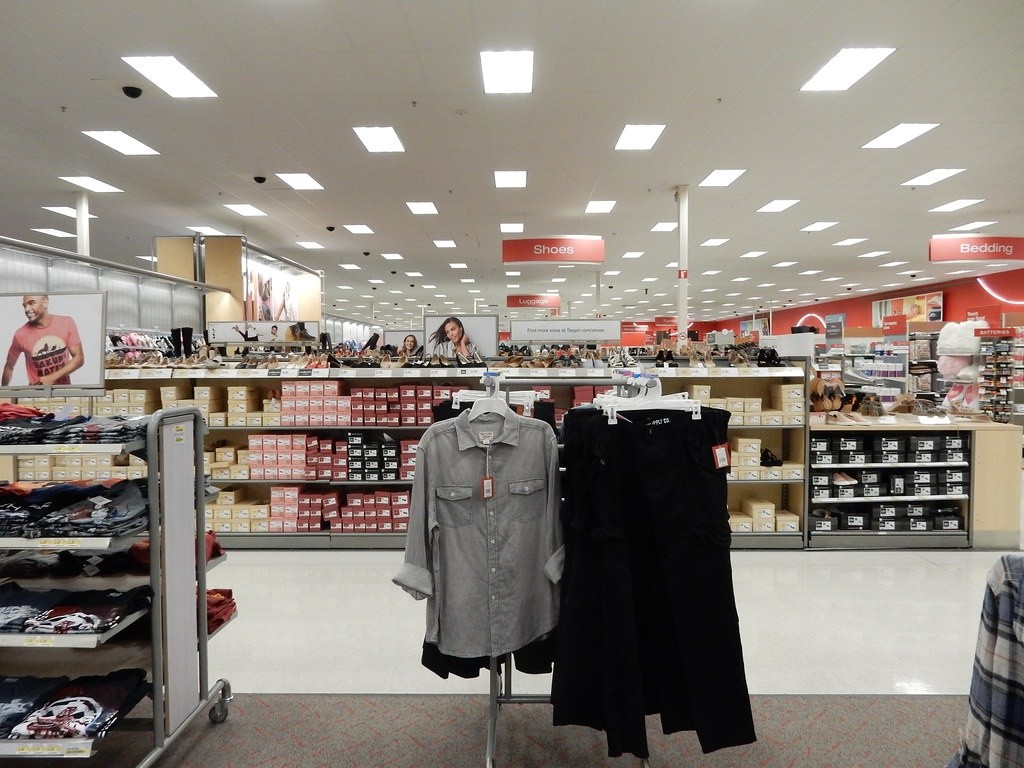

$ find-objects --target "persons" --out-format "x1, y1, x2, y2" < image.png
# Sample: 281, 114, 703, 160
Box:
269, 325, 278, 341
398, 334, 417, 356
275, 282, 295, 322
2, 296, 84, 386
429, 317, 479, 358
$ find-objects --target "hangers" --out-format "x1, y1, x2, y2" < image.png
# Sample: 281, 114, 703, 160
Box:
452, 372, 550, 424
592, 373, 702, 425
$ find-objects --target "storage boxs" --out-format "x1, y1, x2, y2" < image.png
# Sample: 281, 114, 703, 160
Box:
681, 384, 805, 533
809, 436, 969, 532
533, 386, 614, 427
0, 381, 467, 535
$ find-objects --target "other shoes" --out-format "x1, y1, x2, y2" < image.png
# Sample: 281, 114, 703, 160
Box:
809, 377, 975, 426
759, 448, 783, 467
274, 389, 281, 399
267, 389, 274, 401
103, 326, 788, 369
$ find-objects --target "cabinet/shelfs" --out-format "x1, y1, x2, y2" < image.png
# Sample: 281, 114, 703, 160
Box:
811, 421, 1023, 552
814, 332, 1024, 417
101, 368, 811, 550
0, 406, 234, 768
